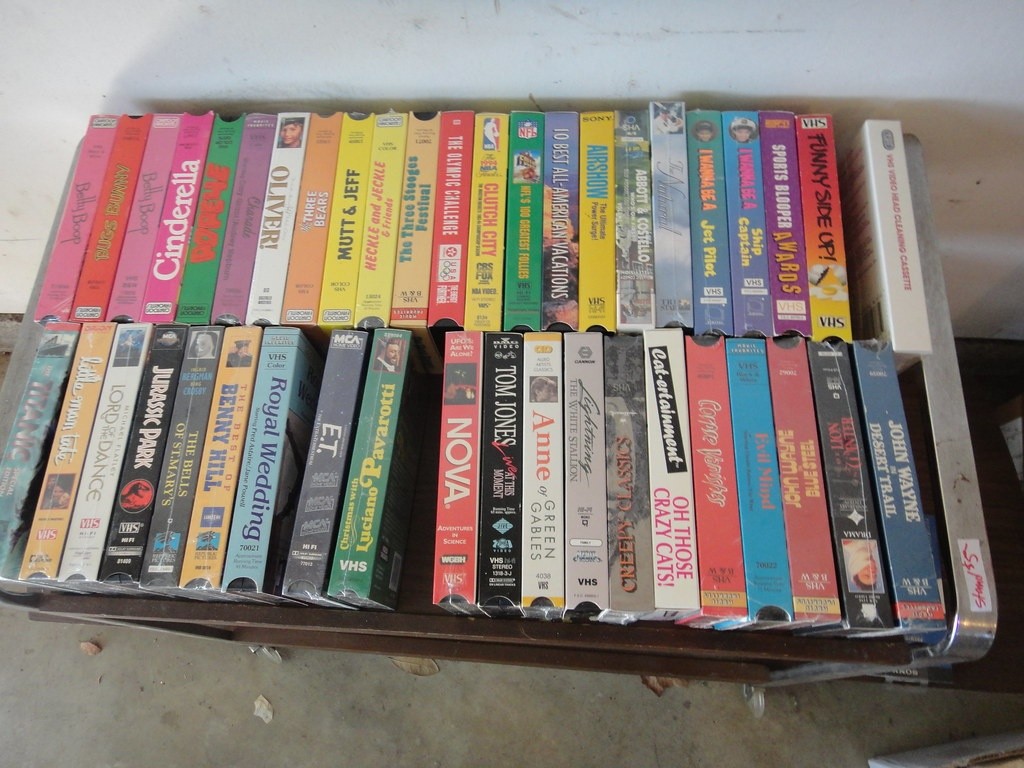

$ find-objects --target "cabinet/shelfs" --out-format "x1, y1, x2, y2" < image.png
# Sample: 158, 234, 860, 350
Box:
1, 128, 1024, 697
